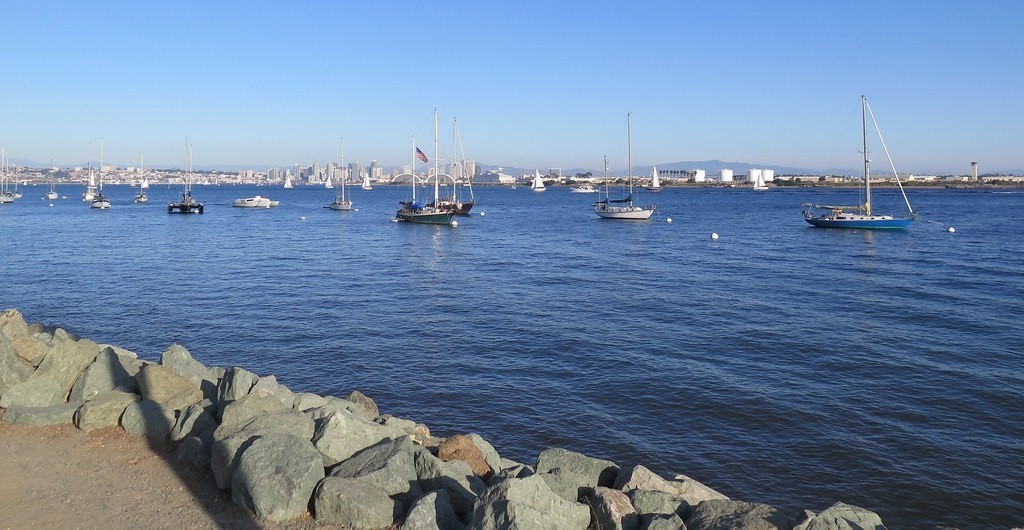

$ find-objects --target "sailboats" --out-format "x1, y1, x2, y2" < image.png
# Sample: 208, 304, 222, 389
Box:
361, 172, 373, 190
135, 154, 147, 202
591, 112, 657, 219
91, 140, 111, 209
0, 147, 99, 204
325, 176, 334, 189
754, 174, 768, 190
142, 178, 150, 190
329, 137, 353, 211
645, 166, 660, 191
531, 170, 546, 192
396, 107, 456, 225
438, 116, 475, 215
284, 178, 293, 189
167, 136, 204, 214
802, 94, 918, 229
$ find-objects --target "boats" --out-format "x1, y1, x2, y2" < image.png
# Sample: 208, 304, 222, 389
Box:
510, 186, 516, 190
130, 182, 139, 190
571, 183, 598, 193
232, 195, 280, 208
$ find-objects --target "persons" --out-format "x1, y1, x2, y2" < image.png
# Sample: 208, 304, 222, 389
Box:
822, 215, 828, 220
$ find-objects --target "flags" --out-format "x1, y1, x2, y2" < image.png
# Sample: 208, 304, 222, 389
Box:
416, 147, 428, 163
